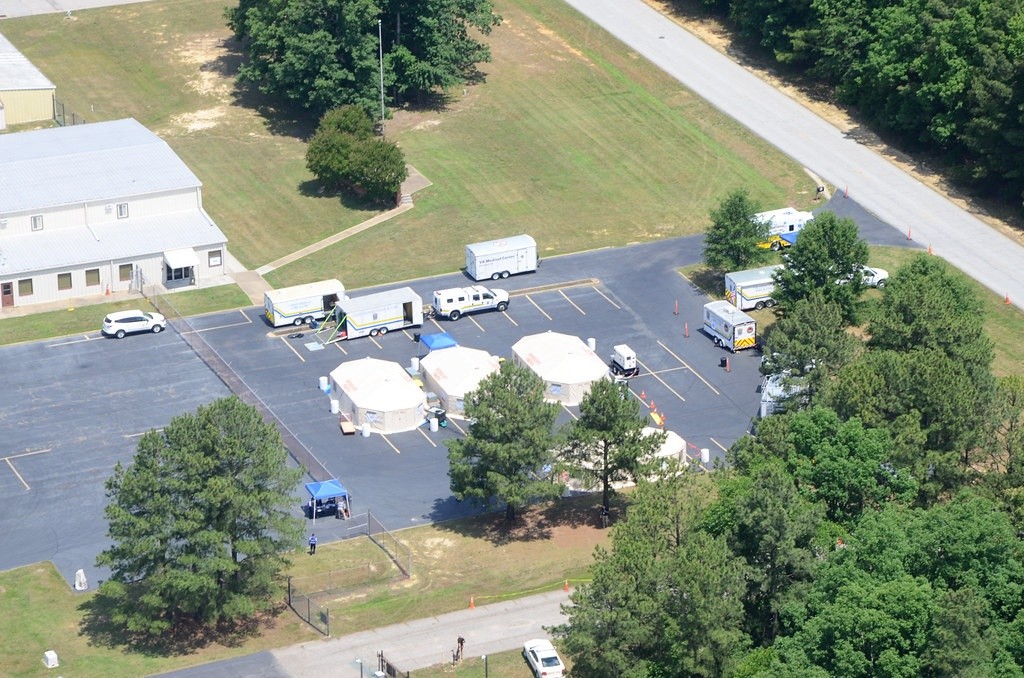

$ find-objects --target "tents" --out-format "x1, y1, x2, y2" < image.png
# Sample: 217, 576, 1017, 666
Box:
420, 346, 511, 421
329, 357, 431, 434
544, 425, 687, 492
418, 332, 458, 361
513, 331, 614, 407
305, 479, 352, 525
779, 229, 801, 260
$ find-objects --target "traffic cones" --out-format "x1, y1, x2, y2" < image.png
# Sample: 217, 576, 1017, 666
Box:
564, 580, 569, 592
468, 597, 475, 609
1003, 292, 1011, 305
661, 414, 666, 422
641, 388, 644, 399
649, 400, 655, 408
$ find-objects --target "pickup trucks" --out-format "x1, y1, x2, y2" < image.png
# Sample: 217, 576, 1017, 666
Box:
432, 284, 509, 321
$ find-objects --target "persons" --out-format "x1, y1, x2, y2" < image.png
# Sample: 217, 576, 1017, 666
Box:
455, 634, 465, 661
308, 533, 318, 555
306, 493, 352, 520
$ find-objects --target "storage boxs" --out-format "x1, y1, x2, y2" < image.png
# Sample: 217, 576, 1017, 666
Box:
406, 368, 446, 423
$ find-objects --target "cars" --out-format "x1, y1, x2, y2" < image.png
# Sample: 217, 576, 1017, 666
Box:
522, 638, 568, 678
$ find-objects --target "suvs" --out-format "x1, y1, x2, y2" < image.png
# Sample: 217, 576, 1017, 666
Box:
837, 266, 889, 291
101, 308, 166, 339
